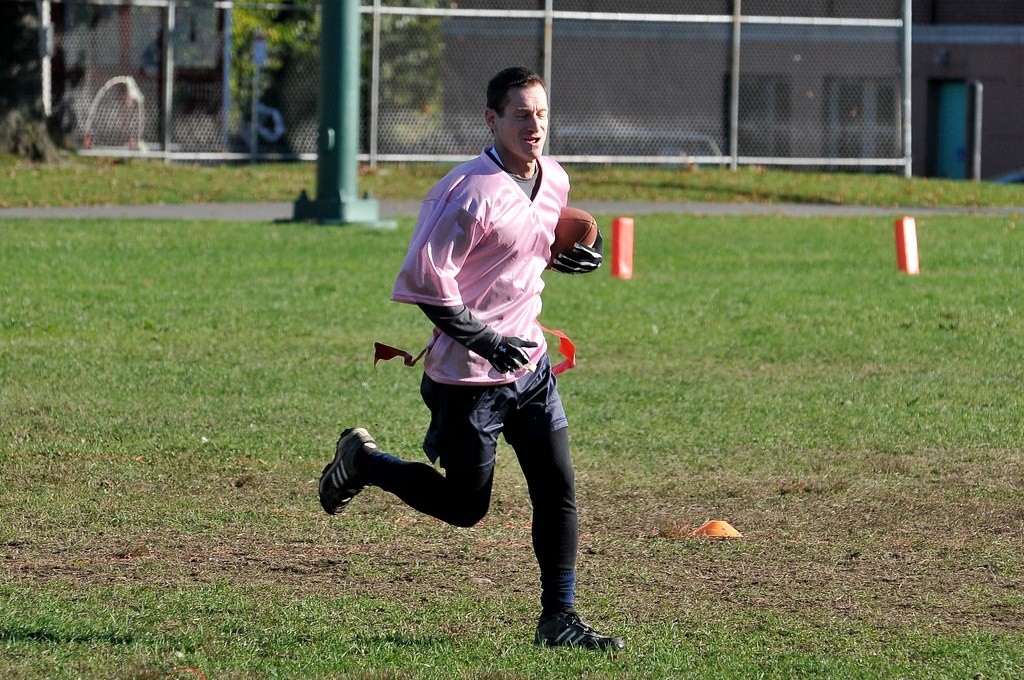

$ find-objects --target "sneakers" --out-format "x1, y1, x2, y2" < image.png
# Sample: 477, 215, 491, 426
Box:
534, 608, 625, 650
317, 426, 377, 515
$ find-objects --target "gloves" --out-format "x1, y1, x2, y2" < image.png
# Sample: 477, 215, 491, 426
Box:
489, 336, 538, 373
552, 229, 603, 275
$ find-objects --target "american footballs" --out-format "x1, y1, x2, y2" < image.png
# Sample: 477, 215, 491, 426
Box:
544, 206, 597, 270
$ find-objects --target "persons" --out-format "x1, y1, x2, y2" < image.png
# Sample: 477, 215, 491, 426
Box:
316, 67, 624, 652
